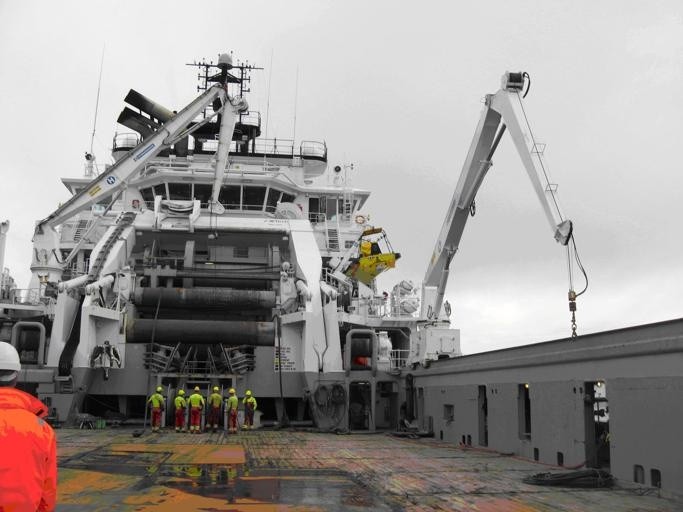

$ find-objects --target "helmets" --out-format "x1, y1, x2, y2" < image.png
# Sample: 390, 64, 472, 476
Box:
156, 386, 162, 392
245, 390, 252, 396
228, 389, 235, 394
213, 386, 219, 391
178, 390, 185, 395
0, 342, 22, 372
193, 386, 200, 390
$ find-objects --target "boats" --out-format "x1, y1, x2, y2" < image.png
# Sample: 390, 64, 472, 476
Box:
342, 227, 401, 286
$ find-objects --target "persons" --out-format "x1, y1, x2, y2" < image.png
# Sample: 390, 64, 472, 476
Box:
173, 389, 186, 433
147, 386, 164, 432
225, 388, 239, 434
0, 341, 60, 512
242, 390, 257, 431
206, 386, 223, 432
188, 386, 205, 434
352, 281, 358, 298
